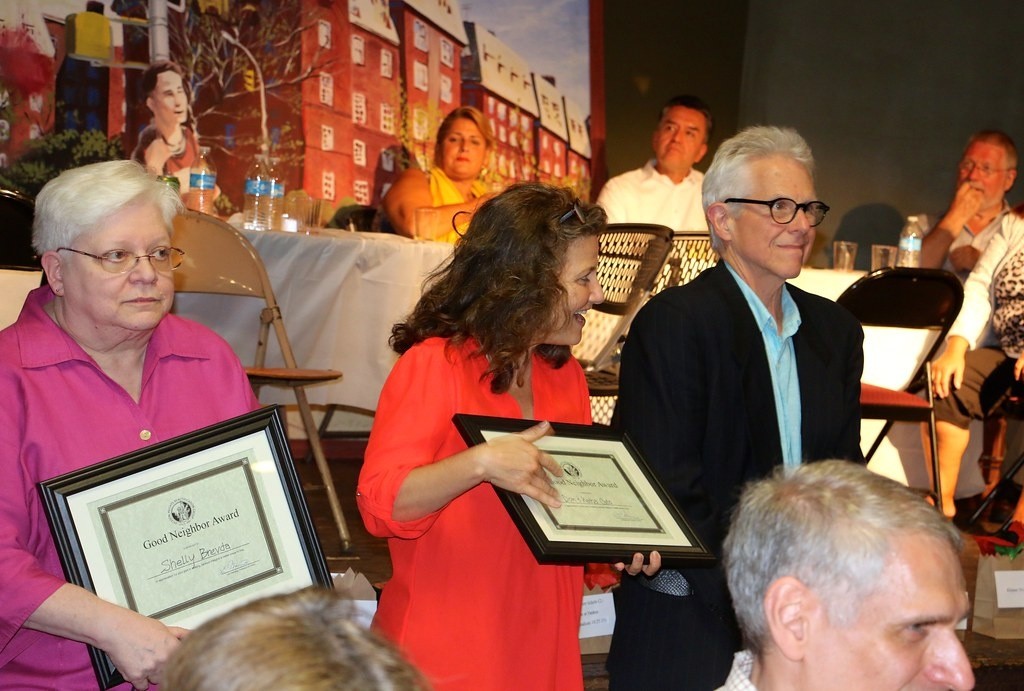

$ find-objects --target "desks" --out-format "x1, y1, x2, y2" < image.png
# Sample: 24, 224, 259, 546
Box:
173, 225, 455, 460
787, 267, 987, 500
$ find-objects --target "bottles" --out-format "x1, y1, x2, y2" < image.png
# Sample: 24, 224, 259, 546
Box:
242, 154, 269, 230
897, 216, 924, 267
261, 158, 285, 232
188, 146, 216, 212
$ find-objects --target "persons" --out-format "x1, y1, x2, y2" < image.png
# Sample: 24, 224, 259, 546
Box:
604, 126, 865, 691
920, 198, 1024, 521
708, 453, 975, 690
355, 177, 662, 691
370, 105, 510, 245
920, 129, 1024, 359
0, 157, 265, 691
159, 583, 432, 691
596, 95, 715, 231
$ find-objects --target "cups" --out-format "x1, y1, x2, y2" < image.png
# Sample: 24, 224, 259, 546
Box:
871, 245, 897, 273
834, 241, 858, 269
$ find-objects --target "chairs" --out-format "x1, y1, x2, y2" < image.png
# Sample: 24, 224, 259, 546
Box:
573, 223, 719, 397
170, 208, 353, 553
834, 268, 1024, 536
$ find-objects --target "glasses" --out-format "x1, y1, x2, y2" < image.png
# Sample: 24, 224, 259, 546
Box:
724, 198, 829, 227
558, 201, 586, 226
958, 160, 1009, 177
56, 247, 186, 274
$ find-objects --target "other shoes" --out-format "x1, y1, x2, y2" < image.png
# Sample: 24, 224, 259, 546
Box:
991, 486, 1017, 523
